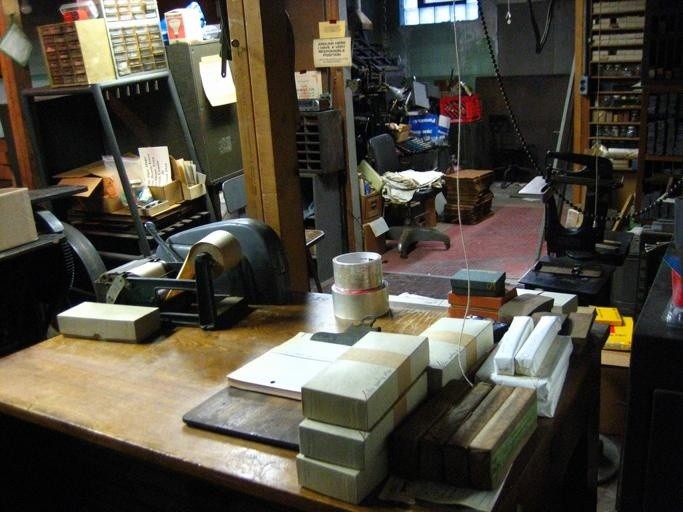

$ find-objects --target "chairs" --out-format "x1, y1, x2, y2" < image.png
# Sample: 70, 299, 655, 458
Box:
223, 173, 325, 292
368, 134, 450, 259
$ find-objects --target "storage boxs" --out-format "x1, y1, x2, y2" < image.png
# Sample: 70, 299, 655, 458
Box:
600, 349, 631, 431
52, 151, 139, 213
0, 186, 38, 254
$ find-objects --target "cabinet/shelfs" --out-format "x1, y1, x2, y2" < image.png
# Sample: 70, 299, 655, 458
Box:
296, 108, 345, 284
572, 0, 683, 222
100, 40, 244, 186
23, 71, 215, 262
74, 0, 170, 80
35, 18, 116, 89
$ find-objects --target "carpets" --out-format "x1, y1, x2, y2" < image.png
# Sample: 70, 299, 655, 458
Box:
382, 205, 547, 280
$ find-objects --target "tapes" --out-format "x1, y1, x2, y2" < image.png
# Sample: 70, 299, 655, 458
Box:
331, 251, 390, 320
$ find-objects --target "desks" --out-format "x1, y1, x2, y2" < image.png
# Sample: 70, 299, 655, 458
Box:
0, 304, 612, 512
634, 224, 672, 319
518, 230, 634, 305
0, 232, 65, 338
615, 240, 683, 512
